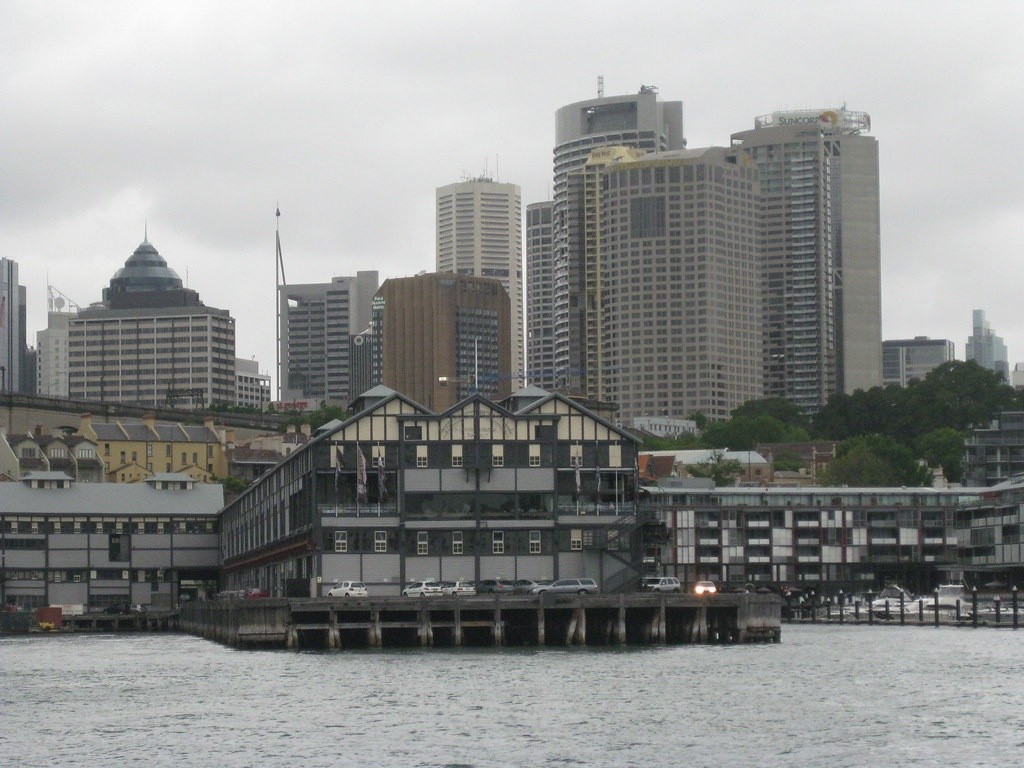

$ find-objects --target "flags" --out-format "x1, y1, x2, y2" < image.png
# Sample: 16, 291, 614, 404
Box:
334, 447, 349, 494
378, 450, 386, 500
357, 447, 368, 499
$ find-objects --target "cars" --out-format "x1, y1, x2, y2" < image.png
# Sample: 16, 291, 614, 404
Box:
474, 579, 516, 597
527, 577, 599, 597
245, 588, 269, 600
402, 581, 444, 597
103, 603, 130, 614
440, 581, 477, 597
128, 604, 147, 616
694, 581, 717, 596
1, 603, 24, 612
510, 579, 550, 595
327, 581, 368, 598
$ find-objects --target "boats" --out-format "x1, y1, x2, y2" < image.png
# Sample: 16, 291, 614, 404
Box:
829, 605, 850, 620
864, 583, 916, 619
846, 605, 870, 619
966, 607, 1024, 623
895, 584, 973, 621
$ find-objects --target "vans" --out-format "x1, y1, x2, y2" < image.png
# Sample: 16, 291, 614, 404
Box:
641, 576, 681, 594
